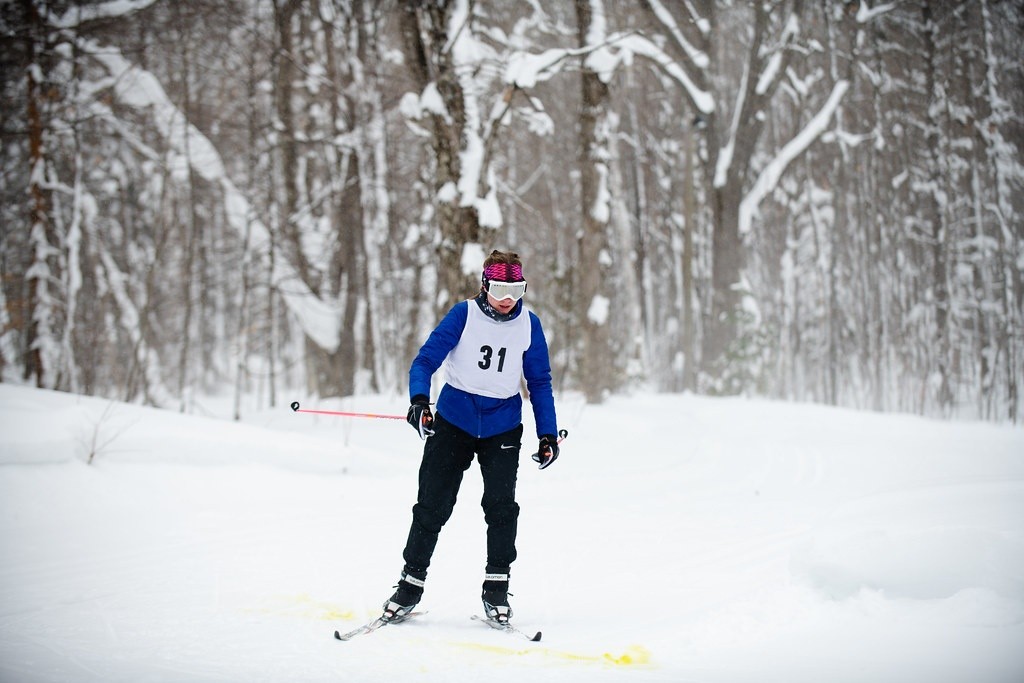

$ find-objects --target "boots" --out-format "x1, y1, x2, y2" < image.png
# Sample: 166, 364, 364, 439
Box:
384, 567, 427, 625
481, 567, 511, 623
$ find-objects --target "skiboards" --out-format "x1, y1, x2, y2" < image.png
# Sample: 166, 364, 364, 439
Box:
333, 608, 543, 643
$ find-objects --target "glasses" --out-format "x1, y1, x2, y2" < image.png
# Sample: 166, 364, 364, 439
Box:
486, 280, 526, 302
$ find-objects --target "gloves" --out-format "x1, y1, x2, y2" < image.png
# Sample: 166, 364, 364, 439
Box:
532, 435, 560, 470
407, 400, 435, 440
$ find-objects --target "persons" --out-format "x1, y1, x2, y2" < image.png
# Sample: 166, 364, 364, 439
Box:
382, 250, 559, 624
